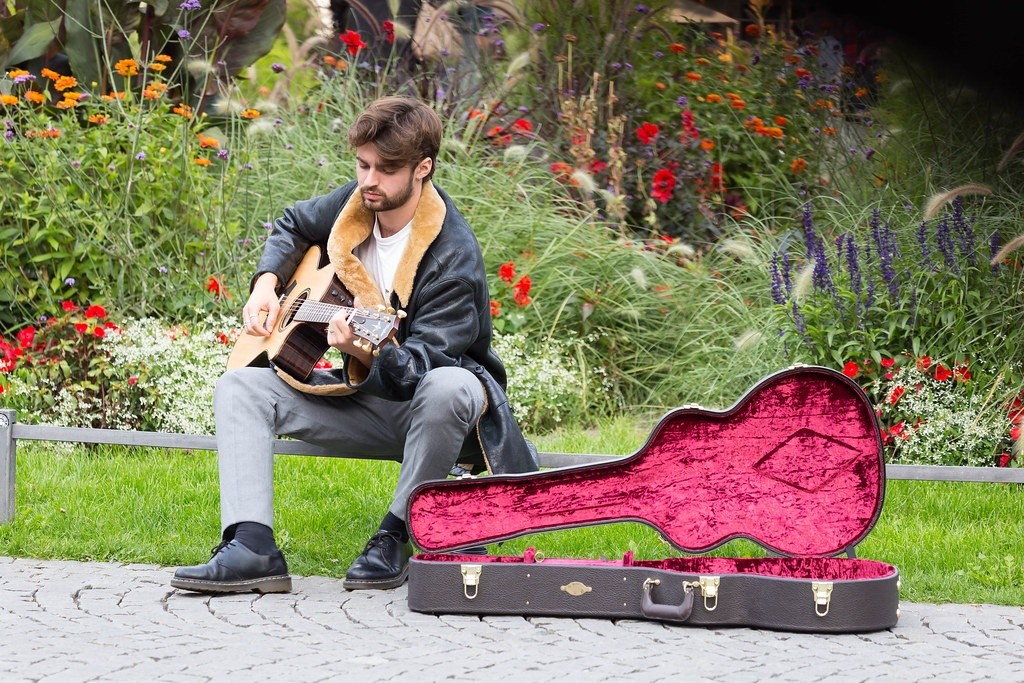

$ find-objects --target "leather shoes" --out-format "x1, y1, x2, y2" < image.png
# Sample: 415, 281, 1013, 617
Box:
171, 538, 292, 593
343, 530, 414, 589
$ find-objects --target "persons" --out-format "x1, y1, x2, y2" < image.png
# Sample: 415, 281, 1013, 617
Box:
166, 95, 545, 595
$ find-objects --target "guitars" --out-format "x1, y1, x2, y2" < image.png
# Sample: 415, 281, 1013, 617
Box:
225, 242, 408, 382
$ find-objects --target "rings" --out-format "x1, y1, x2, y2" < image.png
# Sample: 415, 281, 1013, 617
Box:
329, 330, 334, 333
243, 315, 259, 329
